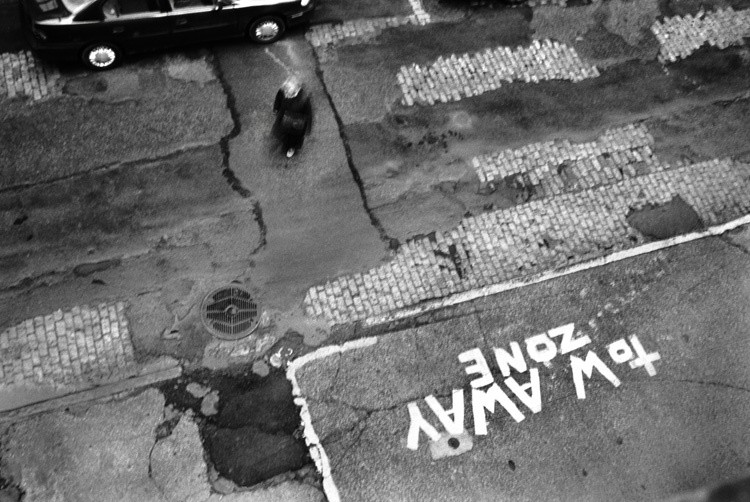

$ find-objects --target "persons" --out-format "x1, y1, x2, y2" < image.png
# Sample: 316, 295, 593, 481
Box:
272, 79, 314, 158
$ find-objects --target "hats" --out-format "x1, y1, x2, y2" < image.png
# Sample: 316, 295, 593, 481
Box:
284, 78, 302, 98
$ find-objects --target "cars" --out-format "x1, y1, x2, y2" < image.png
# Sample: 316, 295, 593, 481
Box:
24, 0, 320, 73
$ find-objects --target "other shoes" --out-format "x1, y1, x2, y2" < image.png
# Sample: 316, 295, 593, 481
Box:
287, 147, 295, 158
